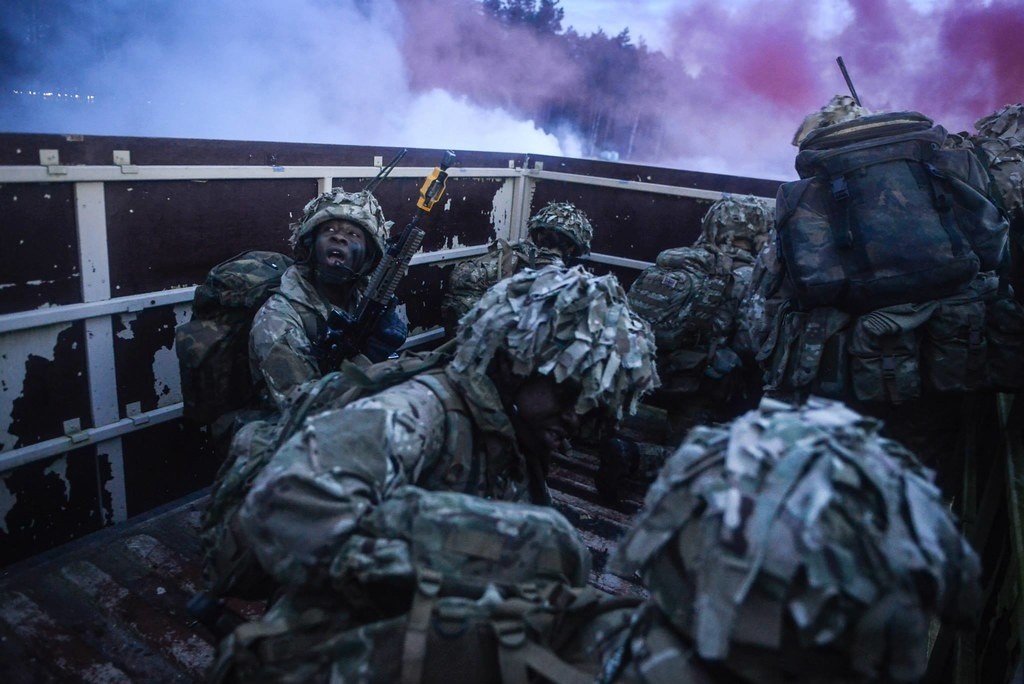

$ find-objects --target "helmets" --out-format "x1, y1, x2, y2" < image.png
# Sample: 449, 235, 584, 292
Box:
614, 393, 988, 663
527, 203, 593, 252
457, 265, 657, 415
293, 189, 392, 259
795, 97, 872, 145
703, 197, 775, 243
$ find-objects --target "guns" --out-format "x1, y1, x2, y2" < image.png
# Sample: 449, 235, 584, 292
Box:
835, 55, 862, 106
310, 147, 462, 369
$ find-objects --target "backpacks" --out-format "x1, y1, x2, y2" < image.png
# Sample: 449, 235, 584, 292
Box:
628, 244, 759, 356
174, 249, 319, 481
439, 240, 555, 344
772, 110, 1011, 313
215, 483, 594, 684
195, 351, 451, 591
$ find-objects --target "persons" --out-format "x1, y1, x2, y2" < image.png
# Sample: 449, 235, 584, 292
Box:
201, 259, 658, 684
505, 199, 595, 279
269, 392, 987, 684
625, 196, 779, 450
179, 187, 407, 476
756, 100, 1024, 683
